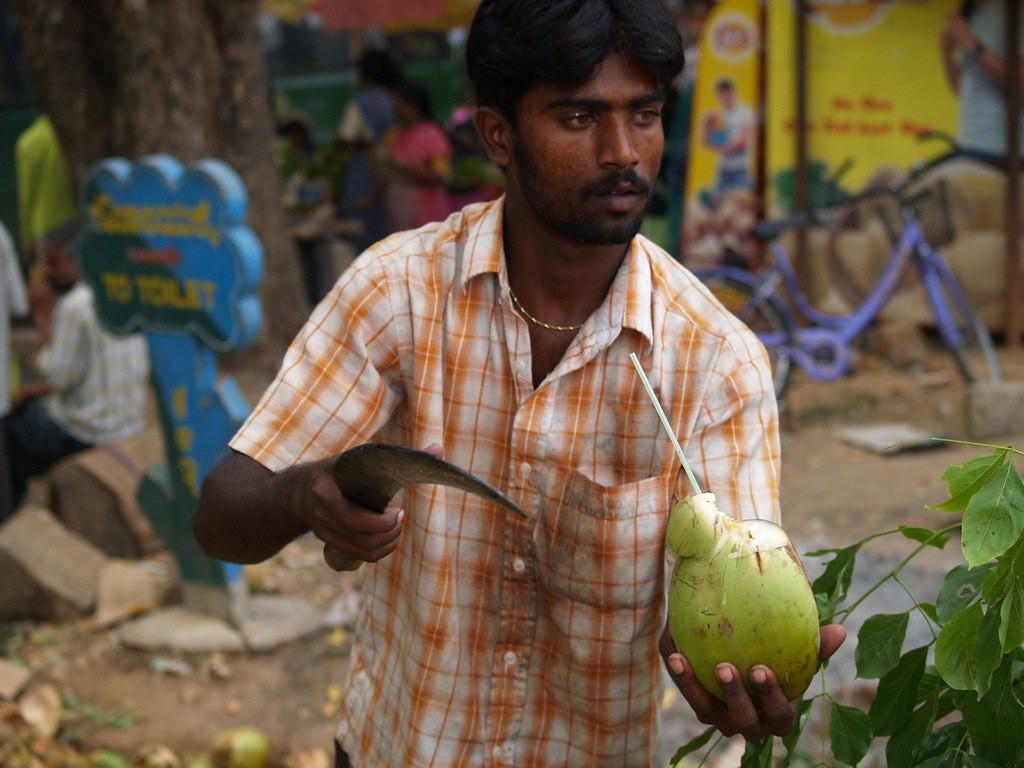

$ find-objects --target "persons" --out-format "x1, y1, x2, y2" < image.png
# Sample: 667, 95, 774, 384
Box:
705, 78, 753, 195
943, 0, 1024, 171
16, 100, 81, 345
268, 43, 499, 243
0, 222, 30, 418
0, 216, 149, 524
193, 1, 847, 768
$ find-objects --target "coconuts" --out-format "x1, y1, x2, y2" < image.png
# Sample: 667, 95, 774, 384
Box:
664, 493, 822, 712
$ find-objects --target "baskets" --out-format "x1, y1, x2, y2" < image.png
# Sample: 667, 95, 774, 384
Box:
875, 177, 955, 254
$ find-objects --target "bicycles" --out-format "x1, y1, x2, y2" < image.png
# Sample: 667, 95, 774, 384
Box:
697, 128, 1008, 406
